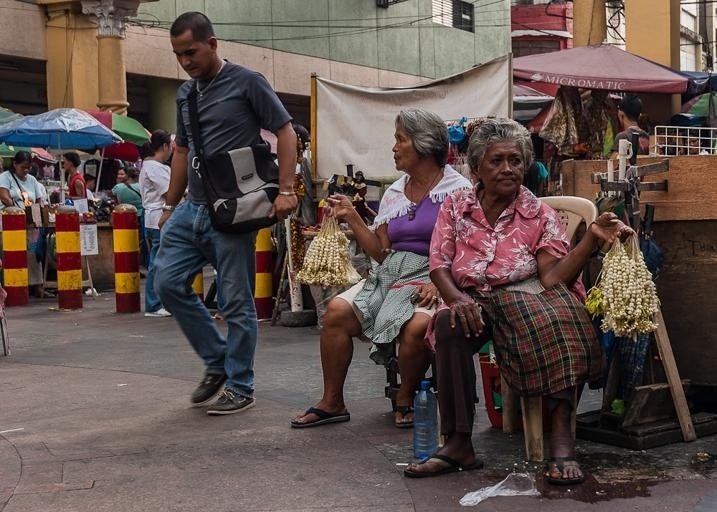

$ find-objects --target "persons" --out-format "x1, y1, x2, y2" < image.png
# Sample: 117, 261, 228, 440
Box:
293, 109, 480, 430
612, 89, 716, 160
153, 10, 299, 411
270, 124, 312, 305
403, 116, 637, 484
0, 130, 189, 319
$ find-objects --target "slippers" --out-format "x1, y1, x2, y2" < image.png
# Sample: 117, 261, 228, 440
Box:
292, 407, 349, 429
404, 452, 482, 476
392, 404, 417, 429
546, 455, 586, 484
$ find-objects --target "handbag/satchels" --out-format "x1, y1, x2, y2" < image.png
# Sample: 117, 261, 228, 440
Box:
193, 141, 284, 233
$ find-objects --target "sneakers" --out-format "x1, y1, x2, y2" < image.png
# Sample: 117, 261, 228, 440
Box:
208, 389, 255, 414
145, 308, 171, 318
189, 369, 222, 408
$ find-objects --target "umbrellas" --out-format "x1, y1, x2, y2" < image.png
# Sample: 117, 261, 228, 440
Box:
0, 109, 25, 130
82, 109, 151, 193
511, 35, 697, 98
0, 140, 58, 167
0, 108, 126, 204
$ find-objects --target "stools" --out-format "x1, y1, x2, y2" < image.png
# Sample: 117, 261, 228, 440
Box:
41, 229, 96, 301
385, 325, 441, 421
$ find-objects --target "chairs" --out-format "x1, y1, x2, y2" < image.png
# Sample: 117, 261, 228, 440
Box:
438, 195, 598, 460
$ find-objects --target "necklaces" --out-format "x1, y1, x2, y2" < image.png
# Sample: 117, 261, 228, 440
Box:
407, 168, 441, 221
196, 60, 225, 97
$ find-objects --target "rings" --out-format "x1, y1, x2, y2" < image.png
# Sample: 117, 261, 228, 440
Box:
431, 295, 437, 300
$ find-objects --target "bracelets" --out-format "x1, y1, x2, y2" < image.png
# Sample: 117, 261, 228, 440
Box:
277, 189, 297, 197
